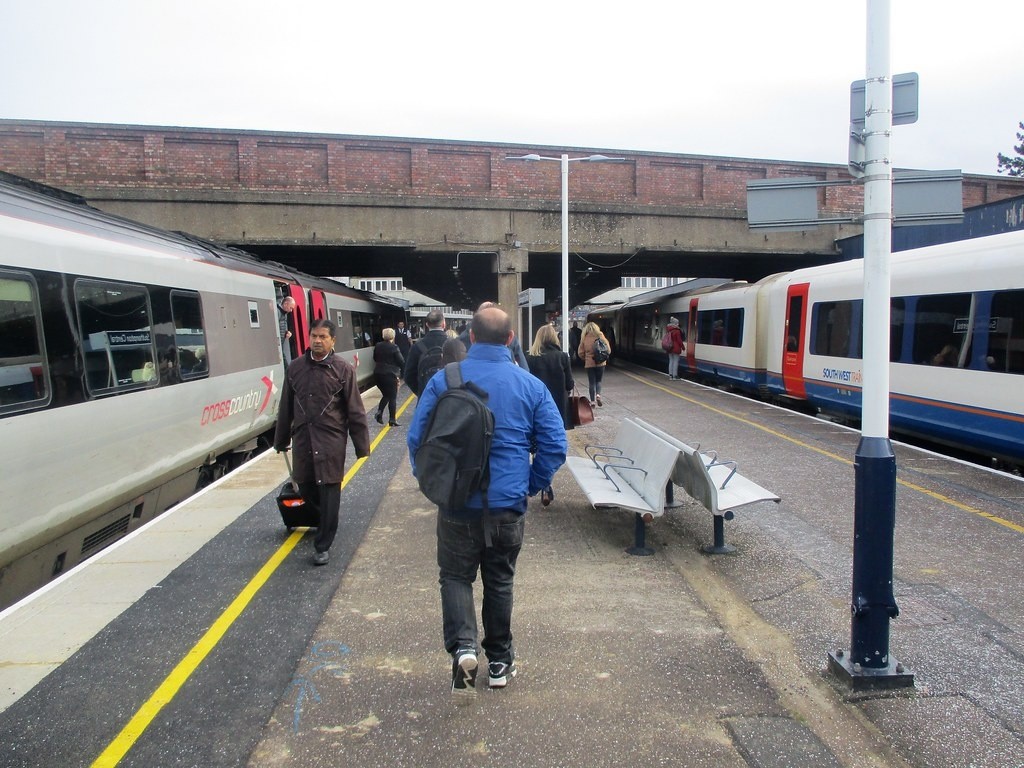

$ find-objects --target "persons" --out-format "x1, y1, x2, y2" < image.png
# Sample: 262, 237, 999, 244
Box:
457, 320, 466, 335
601, 320, 617, 364
667, 316, 685, 381
273, 319, 370, 566
456, 301, 530, 373
578, 322, 611, 408
568, 321, 582, 363
442, 340, 467, 368
353, 326, 372, 347
394, 321, 412, 381
372, 328, 405, 426
191, 348, 207, 373
526, 325, 574, 431
403, 310, 449, 409
407, 307, 568, 709
276, 295, 295, 371
711, 319, 725, 345
932, 346, 958, 368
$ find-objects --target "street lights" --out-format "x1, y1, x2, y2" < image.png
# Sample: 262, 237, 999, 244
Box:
505, 154, 625, 356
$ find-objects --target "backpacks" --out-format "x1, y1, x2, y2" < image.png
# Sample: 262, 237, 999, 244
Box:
416, 337, 453, 394
414, 362, 496, 512
662, 330, 675, 350
593, 335, 608, 364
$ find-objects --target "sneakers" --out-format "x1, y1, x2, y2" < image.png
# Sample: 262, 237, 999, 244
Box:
450, 644, 479, 705
488, 659, 516, 687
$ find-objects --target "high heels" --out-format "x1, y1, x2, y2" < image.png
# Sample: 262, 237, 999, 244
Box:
389, 419, 402, 426
375, 412, 384, 424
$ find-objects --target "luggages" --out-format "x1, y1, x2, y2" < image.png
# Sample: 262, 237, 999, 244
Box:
276, 444, 322, 532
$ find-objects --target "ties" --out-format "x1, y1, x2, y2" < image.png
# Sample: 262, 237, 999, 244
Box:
400, 328, 402, 335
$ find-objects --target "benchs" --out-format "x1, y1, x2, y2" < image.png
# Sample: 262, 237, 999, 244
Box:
565, 418, 685, 555
634, 417, 780, 554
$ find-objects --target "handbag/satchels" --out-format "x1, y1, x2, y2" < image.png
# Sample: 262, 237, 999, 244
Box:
568, 382, 594, 427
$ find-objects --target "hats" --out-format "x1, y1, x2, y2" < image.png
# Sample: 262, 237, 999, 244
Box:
671, 317, 679, 325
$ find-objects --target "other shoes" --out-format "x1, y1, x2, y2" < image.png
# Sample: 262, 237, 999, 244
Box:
596, 396, 602, 406
591, 402, 595, 408
669, 375, 680, 381
312, 550, 329, 564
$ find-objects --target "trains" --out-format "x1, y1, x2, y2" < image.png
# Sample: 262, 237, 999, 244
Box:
583, 230, 1024, 471
0, 182, 410, 612
